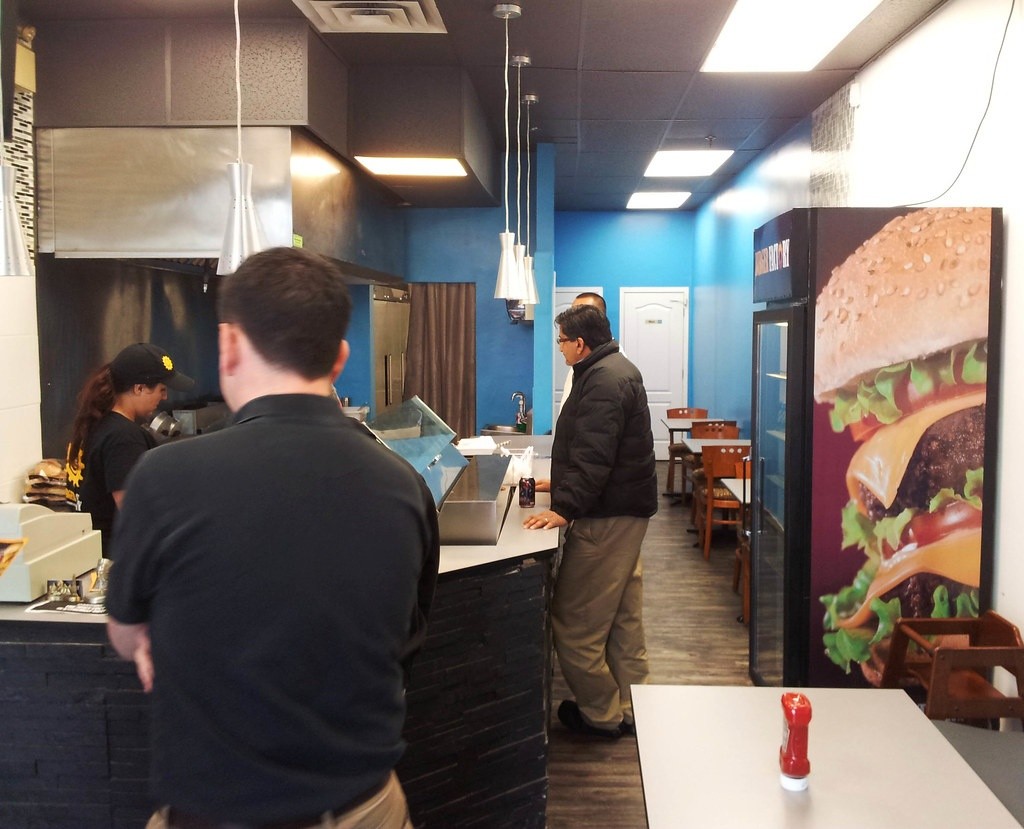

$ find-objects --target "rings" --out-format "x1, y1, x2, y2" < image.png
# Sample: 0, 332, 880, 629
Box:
545, 518, 549, 523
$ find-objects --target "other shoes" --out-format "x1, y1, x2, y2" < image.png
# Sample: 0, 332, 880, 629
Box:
619, 720, 635, 735
557, 700, 625, 739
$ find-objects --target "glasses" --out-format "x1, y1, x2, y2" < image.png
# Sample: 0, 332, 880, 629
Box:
557, 336, 587, 345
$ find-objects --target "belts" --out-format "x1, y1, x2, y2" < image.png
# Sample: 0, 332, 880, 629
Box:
168, 769, 391, 829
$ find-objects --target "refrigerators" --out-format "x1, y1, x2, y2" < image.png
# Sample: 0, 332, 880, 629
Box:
747, 209, 1003, 702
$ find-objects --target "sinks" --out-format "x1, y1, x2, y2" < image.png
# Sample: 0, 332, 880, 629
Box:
480, 423, 528, 436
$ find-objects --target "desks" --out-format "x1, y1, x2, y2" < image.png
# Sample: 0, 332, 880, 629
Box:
660, 418, 725, 506
680, 438, 751, 548
720, 478, 752, 504
630, 683, 1024, 829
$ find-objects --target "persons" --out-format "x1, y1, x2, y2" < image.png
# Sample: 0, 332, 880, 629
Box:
524, 305, 658, 738
559, 292, 628, 409
104, 247, 440, 829
69, 343, 194, 558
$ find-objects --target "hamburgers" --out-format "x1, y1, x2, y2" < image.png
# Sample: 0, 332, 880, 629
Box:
812, 207, 992, 691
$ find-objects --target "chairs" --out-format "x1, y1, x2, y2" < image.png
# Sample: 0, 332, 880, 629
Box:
880, 609, 1024, 729
662, 407, 756, 626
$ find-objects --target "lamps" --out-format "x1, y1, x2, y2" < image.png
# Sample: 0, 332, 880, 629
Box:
216, 0, 271, 276
493, 4, 540, 305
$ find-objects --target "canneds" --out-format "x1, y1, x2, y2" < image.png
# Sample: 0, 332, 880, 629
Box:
518, 476, 536, 508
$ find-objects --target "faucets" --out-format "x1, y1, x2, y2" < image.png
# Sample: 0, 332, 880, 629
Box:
510, 391, 527, 433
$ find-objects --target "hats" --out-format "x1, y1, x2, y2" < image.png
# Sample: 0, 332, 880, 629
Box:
109, 342, 195, 392
